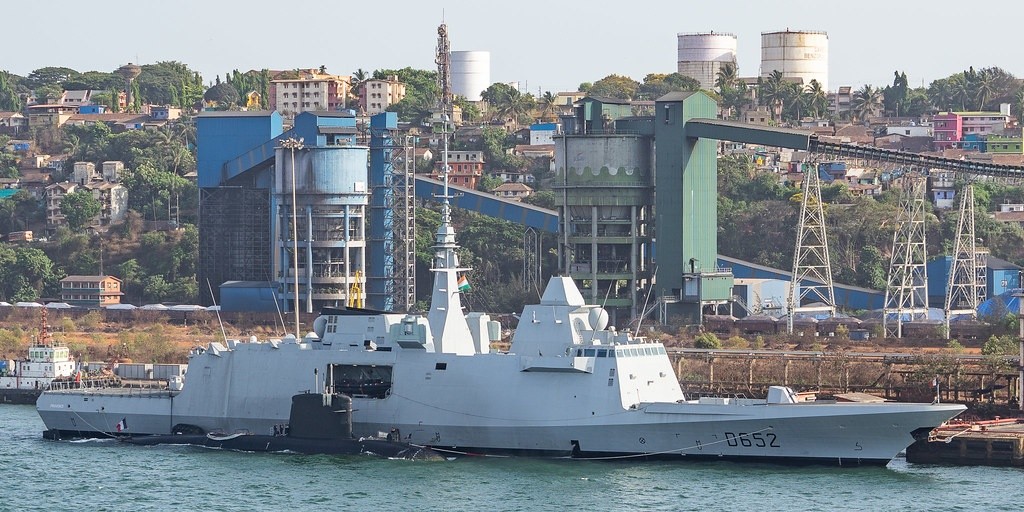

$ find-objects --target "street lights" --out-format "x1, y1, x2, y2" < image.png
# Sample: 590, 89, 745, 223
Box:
279, 137, 307, 338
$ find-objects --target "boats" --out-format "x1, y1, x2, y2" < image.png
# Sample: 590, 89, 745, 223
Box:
34, 115, 968, 468
0, 304, 127, 406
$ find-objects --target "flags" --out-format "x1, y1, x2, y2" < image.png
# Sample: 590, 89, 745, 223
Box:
457, 275, 470, 292
116, 417, 128, 432
